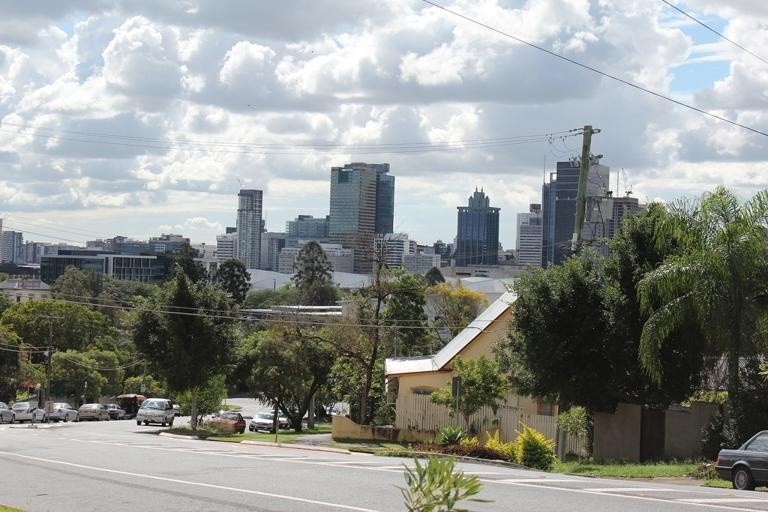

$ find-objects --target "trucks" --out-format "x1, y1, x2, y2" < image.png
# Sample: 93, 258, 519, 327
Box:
117, 393, 147, 420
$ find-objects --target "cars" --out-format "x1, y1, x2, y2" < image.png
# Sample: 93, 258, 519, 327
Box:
12, 402, 46, 423
42, 402, 80, 422
207, 412, 246, 434
0, 401, 16, 424
249, 410, 290, 433
172, 404, 185, 417
103, 404, 126, 420
714, 429, 768, 490
77, 403, 111, 421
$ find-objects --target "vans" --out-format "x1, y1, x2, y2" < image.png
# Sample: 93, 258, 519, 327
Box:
136, 397, 175, 427
